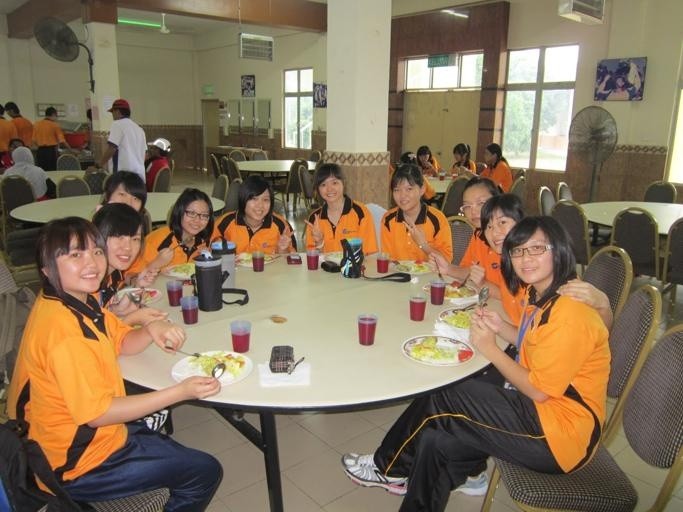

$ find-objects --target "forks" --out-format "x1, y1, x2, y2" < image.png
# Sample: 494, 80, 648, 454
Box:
166, 344, 205, 359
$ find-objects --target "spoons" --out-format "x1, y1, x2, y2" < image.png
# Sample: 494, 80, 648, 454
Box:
476, 286, 491, 319
212, 361, 229, 379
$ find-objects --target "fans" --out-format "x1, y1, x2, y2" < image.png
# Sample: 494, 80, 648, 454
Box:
35, 15, 94, 93
568, 105, 618, 201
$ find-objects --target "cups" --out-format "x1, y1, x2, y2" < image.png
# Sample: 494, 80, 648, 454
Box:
357, 314, 378, 346
231, 321, 252, 353
436, 170, 458, 181
179, 297, 200, 326
409, 297, 425, 322
251, 249, 266, 273
431, 282, 445, 306
163, 281, 186, 307
376, 257, 389, 273
307, 249, 319, 271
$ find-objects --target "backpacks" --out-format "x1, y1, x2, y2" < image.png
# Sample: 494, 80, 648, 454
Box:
0, 419, 98, 512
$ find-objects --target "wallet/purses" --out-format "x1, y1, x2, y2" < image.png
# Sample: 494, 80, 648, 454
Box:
270, 346, 304, 375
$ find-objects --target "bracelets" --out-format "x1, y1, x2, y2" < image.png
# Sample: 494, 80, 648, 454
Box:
417, 240, 428, 249
313, 240, 325, 251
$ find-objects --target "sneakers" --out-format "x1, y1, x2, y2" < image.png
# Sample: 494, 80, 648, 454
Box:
456, 472, 488, 497
342, 452, 409, 496
136, 409, 170, 432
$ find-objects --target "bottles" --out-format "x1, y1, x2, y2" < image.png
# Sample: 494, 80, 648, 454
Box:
194, 256, 223, 311
342, 238, 362, 277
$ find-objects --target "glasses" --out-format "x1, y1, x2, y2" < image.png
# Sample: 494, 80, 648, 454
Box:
184, 211, 209, 221
461, 203, 486, 212
509, 245, 554, 259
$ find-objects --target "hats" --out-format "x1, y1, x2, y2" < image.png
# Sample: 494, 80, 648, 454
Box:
146, 138, 171, 153
106, 99, 130, 112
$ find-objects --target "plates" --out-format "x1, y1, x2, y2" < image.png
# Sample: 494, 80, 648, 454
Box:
422, 280, 481, 303
439, 307, 500, 332
115, 288, 164, 305
402, 333, 478, 368
392, 262, 436, 276
171, 351, 253, 391
235, 252, 278, 267
162, 262, 208, 280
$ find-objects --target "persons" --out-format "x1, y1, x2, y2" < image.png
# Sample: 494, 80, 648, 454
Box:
392, 143, 515, 197
102, 170, 175, 284
0, 95, 92, 205
141, 186, 214, 268
209, 174, 299, 254
446, 175, 506, 271
338, 215, 613, 511
144, 137, 175, 193
4, 216, 223, 512
95, 99, 148, 197
302, 162, 379, 258
379, 164, 453, 265
449, 194, 613, 496
87, 203, 166, 327
